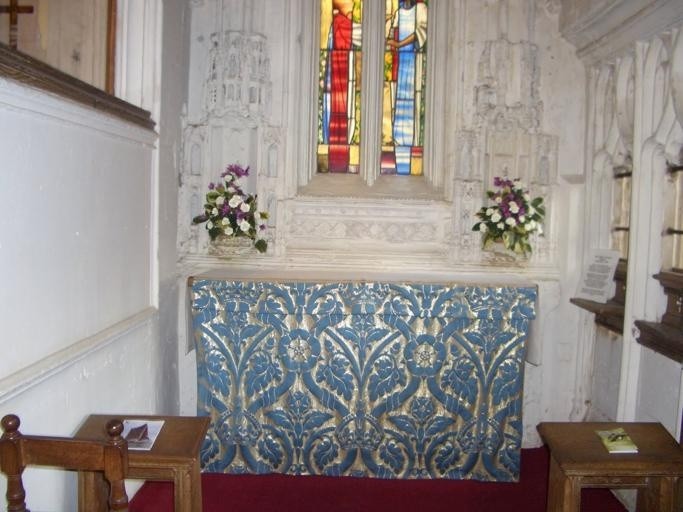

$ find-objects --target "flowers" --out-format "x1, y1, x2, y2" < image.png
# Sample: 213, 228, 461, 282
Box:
193, 166, 271, 258
469, 170, 550, 263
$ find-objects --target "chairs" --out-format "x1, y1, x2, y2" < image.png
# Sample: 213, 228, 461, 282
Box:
2, 413, 129, 510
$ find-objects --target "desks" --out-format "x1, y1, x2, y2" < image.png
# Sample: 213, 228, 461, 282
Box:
72, 413, 209, 512
189, 267, 541, 485
538, 421, 683, 512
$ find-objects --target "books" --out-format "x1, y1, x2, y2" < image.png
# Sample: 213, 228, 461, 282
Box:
596, 427, 639, 454
119, 418, 166, 451
